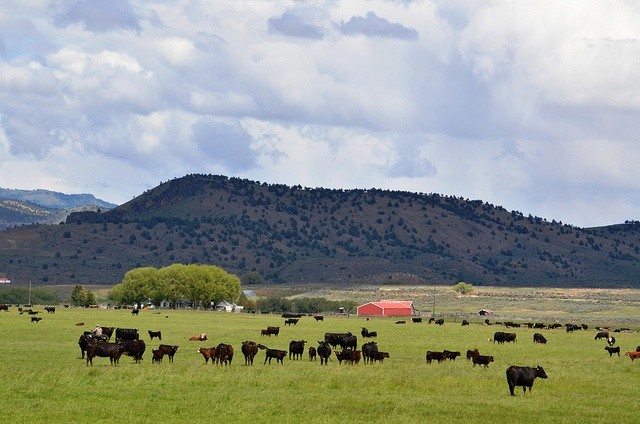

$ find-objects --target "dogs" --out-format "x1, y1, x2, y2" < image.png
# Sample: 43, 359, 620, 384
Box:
466, 348, 480, 361
472, 355, 494, 369
605, 346, 621, 357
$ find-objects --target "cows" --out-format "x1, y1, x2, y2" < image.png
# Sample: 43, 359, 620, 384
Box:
78, 334, 97, 359
533, 332, 548, 345
324, 331, 353, 350
361, 326, 368, 338
84, 329, 109, 344
99, 326, 116, 340
285, 320, 293, 326
189, 332, 209, 342
426, 350, 447, 365
594, 331, 609, 341
86, 338, 124, 367
500, 332, 517, 345
395, 320, 406, 324
130, 309, 140, 316
607, 336, 615, 347
494, 331, 511, 345
119, 333, 139, 342
266, 326, 280, 338
148, 329, 163, 341
241, 340, 258, 366
314, 315, 324, 322
75, 321, 85, 327
365, 331, 378, 339
261, 328, 269, 337
362, 341, 378, 365
317, 340, 332, 366
435, 319, 444, 325
115, 327, 139, 343
334, 350, 354, 366
197, 347, 217, 365
373, 350, 390, 363
288, 318, 299, 326
30, 317, 43, 323
118, 339, 146, 364
214, 343, 234, 367
443, 349, 461, 362
158, 344, 179, 363
505, 365, 548, 396
44, 307, 56, 314
337, 334, 357, 351
151, 348, 164, 364
624, 350, 640, 363
263, 348, 289, 366
342, 349, 362, 365
411, 318, 422, 323
289, 340, 308, 361
308, 347, 317, 361
